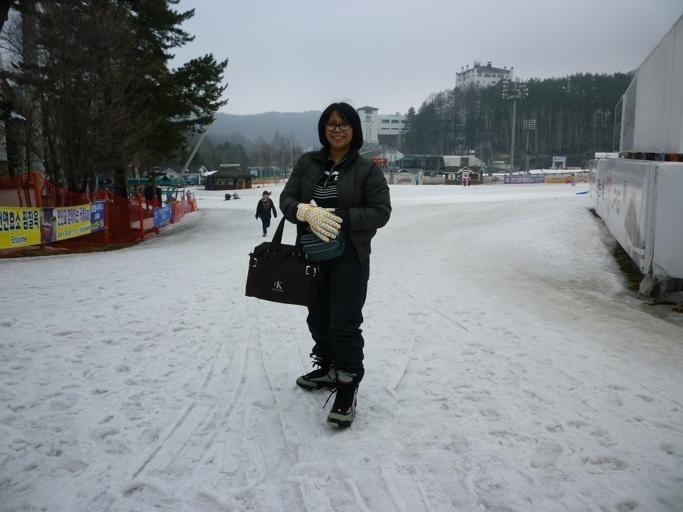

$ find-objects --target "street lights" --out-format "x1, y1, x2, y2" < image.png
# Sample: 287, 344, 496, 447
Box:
502, 80, 529, 170
523, 119, 536, 171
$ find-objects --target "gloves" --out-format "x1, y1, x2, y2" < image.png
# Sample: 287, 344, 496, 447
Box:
296, 199, 342, 242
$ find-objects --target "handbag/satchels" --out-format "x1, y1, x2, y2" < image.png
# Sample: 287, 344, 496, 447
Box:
245, 201, 344, 307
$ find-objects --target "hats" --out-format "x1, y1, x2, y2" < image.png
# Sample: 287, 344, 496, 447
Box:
263, 191, 268, 195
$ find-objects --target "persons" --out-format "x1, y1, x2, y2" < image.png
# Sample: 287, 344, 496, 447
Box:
279, 101, 393, 428
255, 190, 278, 237
127, 179, 194, 210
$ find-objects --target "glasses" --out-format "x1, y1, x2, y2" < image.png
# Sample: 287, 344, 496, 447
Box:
325, 124, 351, 131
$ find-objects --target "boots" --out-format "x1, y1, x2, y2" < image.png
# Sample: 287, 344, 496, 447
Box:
297, 353, 358, 428
